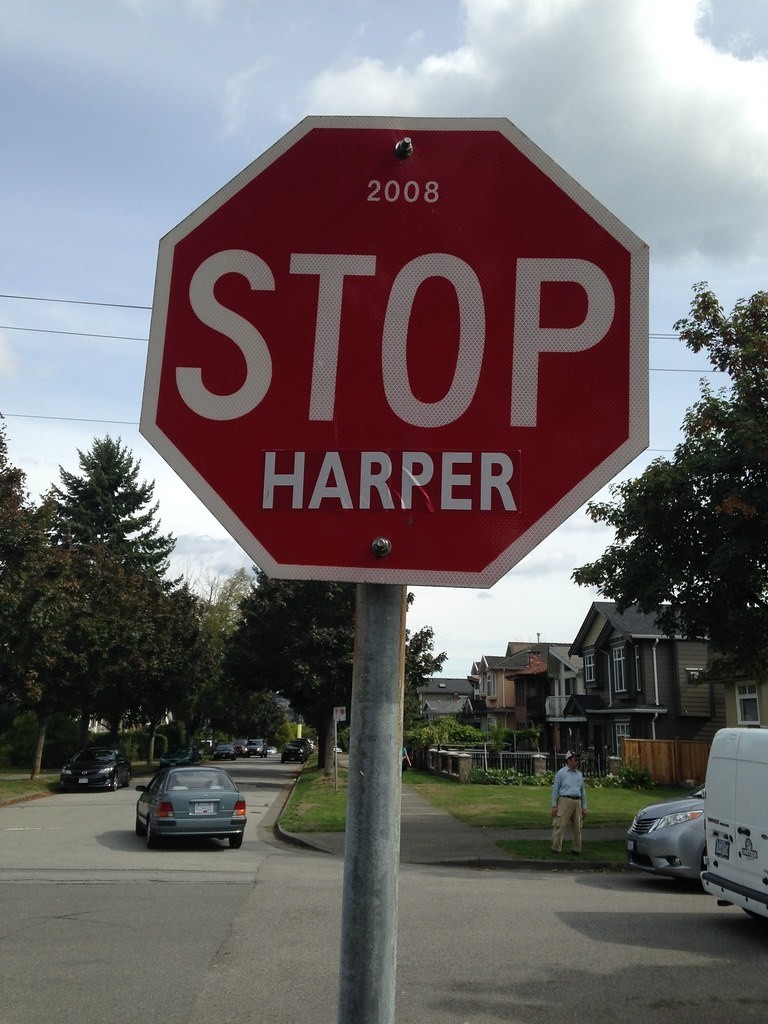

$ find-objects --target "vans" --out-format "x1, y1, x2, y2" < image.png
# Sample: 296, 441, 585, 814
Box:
700, 726, 768, 920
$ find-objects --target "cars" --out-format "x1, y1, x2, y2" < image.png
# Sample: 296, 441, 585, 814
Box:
213, 744, 237, 761
59, 746, 132, 791
242, 739, 268, 758
160, 745, 203, 766
135, 766, 248, 849
626, 783, 708, 880
281, 738, 312, 764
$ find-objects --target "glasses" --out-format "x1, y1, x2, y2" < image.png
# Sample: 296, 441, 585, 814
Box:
569, 758, 579, 762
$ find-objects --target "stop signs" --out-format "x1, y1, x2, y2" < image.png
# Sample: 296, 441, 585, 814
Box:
139, 115, 650, 590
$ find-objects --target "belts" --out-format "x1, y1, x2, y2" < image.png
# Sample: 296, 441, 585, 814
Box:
561, 795, 581, 799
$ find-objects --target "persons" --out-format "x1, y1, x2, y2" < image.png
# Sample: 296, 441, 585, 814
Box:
402, 746, 412, 781
552, 750, 586, 855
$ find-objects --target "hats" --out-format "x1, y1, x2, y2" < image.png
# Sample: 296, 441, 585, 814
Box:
566, 750, 581, 759
